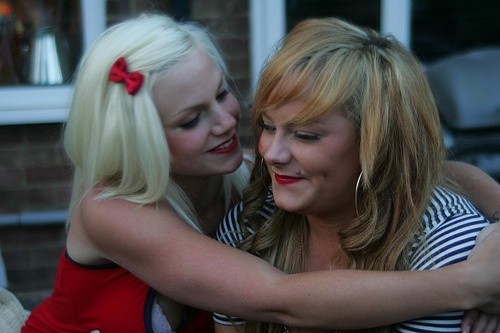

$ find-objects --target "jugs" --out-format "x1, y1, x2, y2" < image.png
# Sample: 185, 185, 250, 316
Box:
26, 26, 65, 85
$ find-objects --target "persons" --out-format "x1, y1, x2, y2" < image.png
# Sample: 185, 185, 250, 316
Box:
215, 18, 490, 333
18, 13, 500, 333
